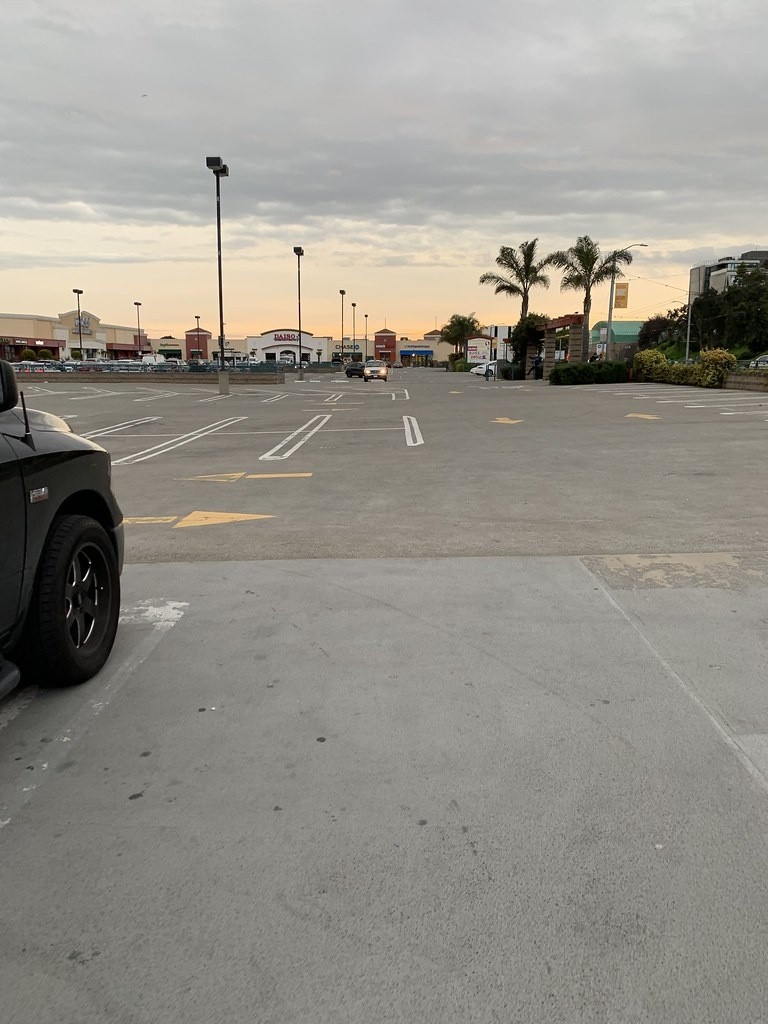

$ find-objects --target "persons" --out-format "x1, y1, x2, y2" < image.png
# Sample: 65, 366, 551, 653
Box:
525, 352, 542, 380
589, 352, 598, 363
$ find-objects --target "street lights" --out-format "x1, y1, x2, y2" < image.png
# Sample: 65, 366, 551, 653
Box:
295, 246, 303, 380
365, 314, 368, 361
340, 290, 344, 372
605, 243, 648, 362
206, 156, 230, 396
134, 302, 142, 358
196, 316, 205, 362
352, 302, 356, 362
73, 288, 83, 359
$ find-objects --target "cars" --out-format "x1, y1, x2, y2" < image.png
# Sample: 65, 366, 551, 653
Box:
386, 361, 403, 368
345, 362, 365, 378
470, 359, 497, 377
9, 358, 345, 382
364, 360, 388, 382
0, 356, 126, 690
